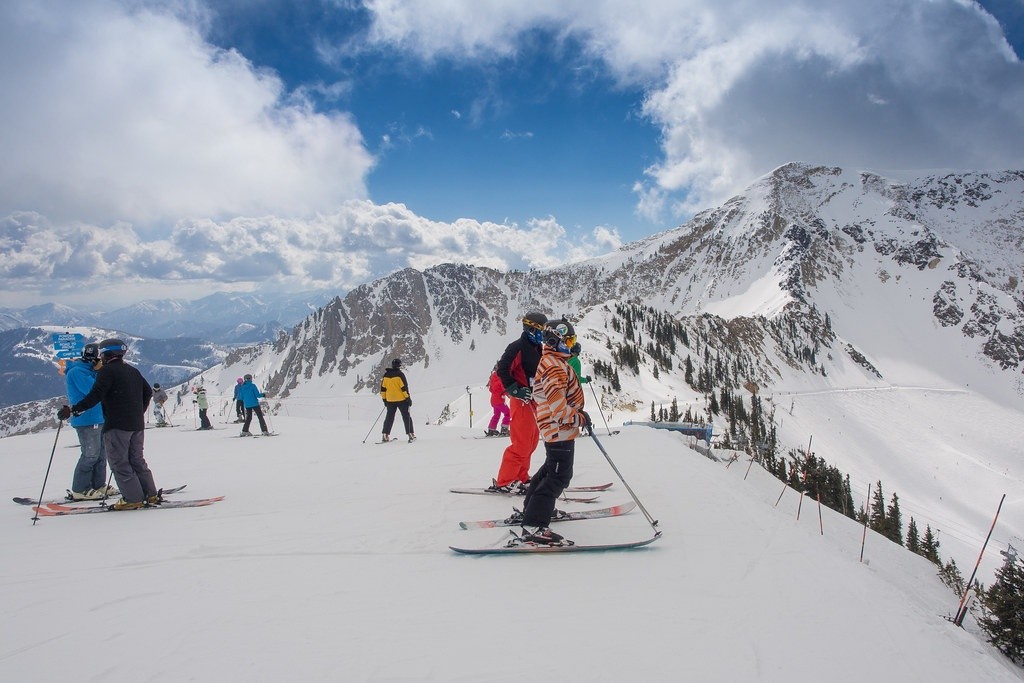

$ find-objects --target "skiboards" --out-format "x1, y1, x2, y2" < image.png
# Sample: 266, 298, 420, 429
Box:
448, 501, 663, 552
232, 431, 278, 438
180, 427, 227, 430
449, 475, 614, 502
31, 494, 226, 516
460, 432, 512, 440
11, 483, 188, 506
375, 437, 417, 444
579, 430, 621, 437
144, 424, 180, 429
220, 420, 248, 424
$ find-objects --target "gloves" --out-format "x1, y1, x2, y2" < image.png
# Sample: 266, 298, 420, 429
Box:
407, 397, 412, 407
58, 404, 70, 420
586, 375, 592, 382
505, 381, 531, 404
579, 410, 592, 428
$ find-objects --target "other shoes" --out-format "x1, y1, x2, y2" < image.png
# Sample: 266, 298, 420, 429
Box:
143, 495, 159, 505
489, 430, 501, 437
114, 497, 146, 510
73, 488, 105, 500
551, 508, 566, 518
382, 434, 389, 441
234, 419, 247, 423
501, 427, 510, 436
242, 431, 253, 436
155, 421, 167, 428
522, 526, 565, 545
98, 483, 116, 494
408, 433, 416, 443
499, 480, 527, 492
199, 426, 213, 430
262, 431, 272, 435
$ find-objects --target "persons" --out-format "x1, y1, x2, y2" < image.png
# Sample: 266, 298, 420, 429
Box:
63, 343, 117, 500
192, 386, 211, 429
568, 342, 592, 383
520, 319, 592, 546
233, 378, 245, 423
238, 374, 268, 435
496, 312, 548, 493
152, 383, 168, 427
57, 339, 157, 510
380, 359, 414, 441
488, 372, 510, 435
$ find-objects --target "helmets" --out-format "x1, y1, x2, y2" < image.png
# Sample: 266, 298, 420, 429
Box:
523, 313, 548, 332
197, 385, 203, 391
99, 339, 127, 365
543, 320, 575, 348
244, 374, 252, 381
154, 383, 159, 388
237, 377, 243, 384
81, 344, 101, 364
392, 359, 401, 368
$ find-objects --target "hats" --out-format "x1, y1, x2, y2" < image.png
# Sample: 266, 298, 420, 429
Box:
571, 342, 581, 354
